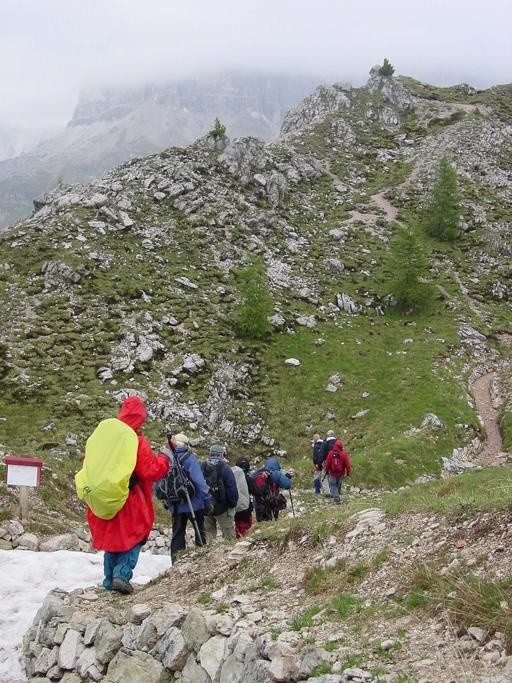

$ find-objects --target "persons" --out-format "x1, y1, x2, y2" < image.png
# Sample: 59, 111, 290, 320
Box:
166, 428, 351, 564
87, 394, 178, 595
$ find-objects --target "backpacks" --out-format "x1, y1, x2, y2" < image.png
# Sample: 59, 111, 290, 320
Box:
247, 468, 278, 505
154, 449, 196, 509
202, 460, 226, 502
330, 449, 344, 477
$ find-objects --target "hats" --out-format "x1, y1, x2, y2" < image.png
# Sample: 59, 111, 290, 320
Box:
174, 433, 189, 443
210, 445, 224, 453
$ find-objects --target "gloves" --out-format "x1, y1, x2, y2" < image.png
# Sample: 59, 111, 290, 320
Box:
207, 502, 215, 517
285, 472, 292, 478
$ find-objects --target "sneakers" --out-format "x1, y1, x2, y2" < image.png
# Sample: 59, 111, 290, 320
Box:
111, 577, 133, 594
333, 501, 341, 504
